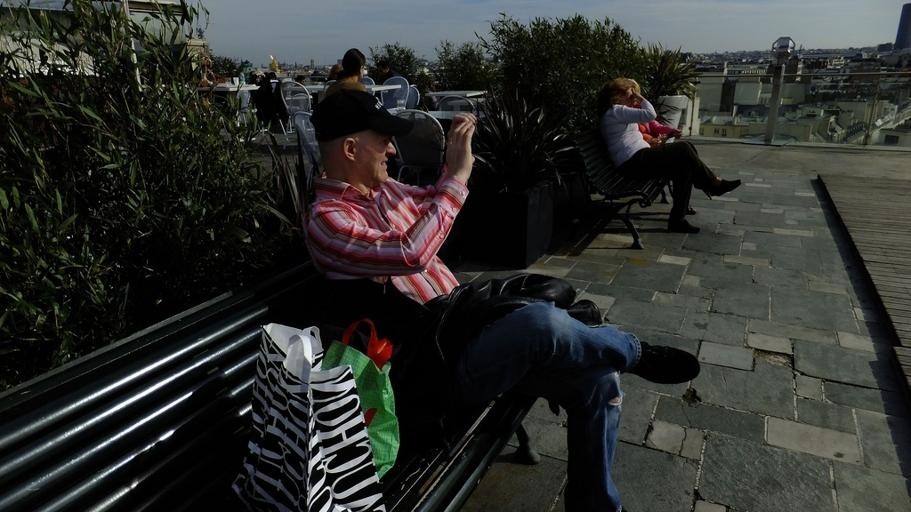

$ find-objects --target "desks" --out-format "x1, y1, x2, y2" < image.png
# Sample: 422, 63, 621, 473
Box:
198, 82, 262, 91
424, 89, 488, 112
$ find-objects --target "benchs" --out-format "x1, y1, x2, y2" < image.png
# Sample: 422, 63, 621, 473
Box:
1, 247, 545, 512
575, 121, 674, 254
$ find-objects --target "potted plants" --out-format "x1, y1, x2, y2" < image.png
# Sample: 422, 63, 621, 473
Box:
648, 40, 703, 144
471, 86, 567, 270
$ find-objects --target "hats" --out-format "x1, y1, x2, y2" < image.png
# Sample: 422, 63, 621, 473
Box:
310, 89, 415, 140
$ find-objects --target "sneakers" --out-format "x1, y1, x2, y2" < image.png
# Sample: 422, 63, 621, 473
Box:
637, 341, 700, 383
668, 221, 699, 234
716, 180, 741, 192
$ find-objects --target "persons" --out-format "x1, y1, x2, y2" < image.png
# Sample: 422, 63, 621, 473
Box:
303, 87, 701, 511
198, 65, 217, 82
249, 70, 288, 135
318, 48, 367, 105
601, 75, 743, 234
291, 74, 305, 95
377, 62, 399, 85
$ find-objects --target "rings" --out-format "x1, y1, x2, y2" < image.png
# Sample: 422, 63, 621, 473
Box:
446, 141, 453, 147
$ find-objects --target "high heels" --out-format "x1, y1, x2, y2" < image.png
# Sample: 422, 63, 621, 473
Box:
704, 181, 726, 200
685, 206, 696, 215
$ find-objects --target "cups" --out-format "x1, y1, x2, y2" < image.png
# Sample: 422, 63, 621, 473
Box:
396, 99, 406, 113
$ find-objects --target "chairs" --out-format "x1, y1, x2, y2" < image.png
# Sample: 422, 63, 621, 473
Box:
278, 74, 476, 193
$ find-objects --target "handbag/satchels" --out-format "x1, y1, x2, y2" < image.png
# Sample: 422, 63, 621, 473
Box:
319, 340, 401, 480
230, 324, 386, 512
